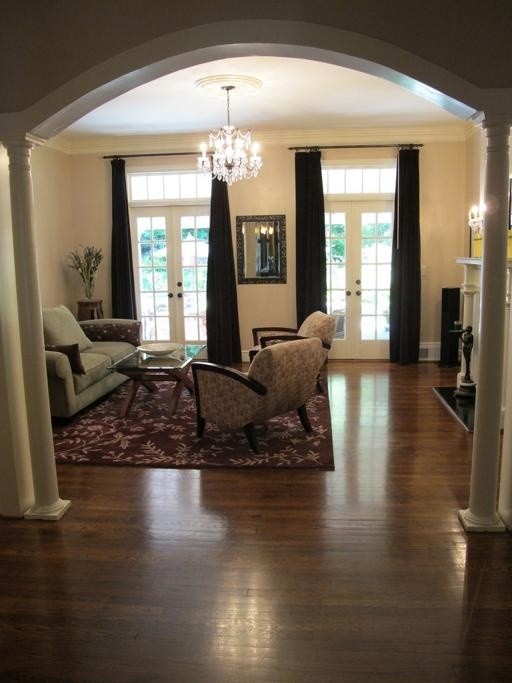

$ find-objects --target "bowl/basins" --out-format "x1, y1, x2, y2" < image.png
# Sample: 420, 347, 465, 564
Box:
137, 358, 183, 366
136, 341, 182, 356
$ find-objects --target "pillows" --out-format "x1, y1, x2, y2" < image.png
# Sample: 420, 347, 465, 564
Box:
44, 343, 86, 376
43, 304, 93, 352
80, 321, 142, 347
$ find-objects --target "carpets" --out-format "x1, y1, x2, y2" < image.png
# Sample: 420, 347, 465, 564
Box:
51, 366, 336, 471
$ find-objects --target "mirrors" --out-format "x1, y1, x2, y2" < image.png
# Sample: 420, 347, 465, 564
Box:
235, 214, 288, 284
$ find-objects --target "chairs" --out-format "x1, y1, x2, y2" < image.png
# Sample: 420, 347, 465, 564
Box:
191, 336, 323, 452
248, 311, 336, 392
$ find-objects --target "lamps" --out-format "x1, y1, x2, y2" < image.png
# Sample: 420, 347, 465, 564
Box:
196, 85, 263, 185
467, 207, 483, 235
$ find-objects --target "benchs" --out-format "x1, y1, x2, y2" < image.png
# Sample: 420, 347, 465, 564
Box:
45, 319, 143, 418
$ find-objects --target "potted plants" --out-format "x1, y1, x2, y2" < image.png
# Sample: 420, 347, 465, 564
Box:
65, 246, 105, 320
